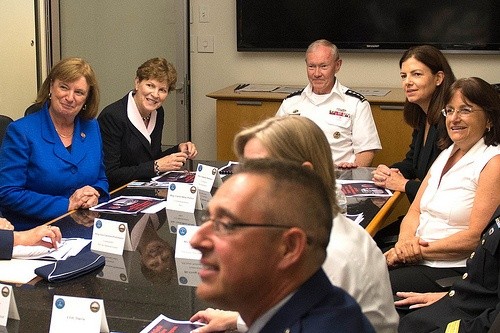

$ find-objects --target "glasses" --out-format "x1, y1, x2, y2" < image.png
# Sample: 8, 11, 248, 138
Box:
441, 106, 490, 117
199, 212, 312, 245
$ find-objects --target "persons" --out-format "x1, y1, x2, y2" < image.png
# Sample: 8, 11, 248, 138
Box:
383, 77, 500, 294
274, 39, 382, 169
0, 58, 110, 230
189, 115, 400, 333
373, 45, 456, 253
97, 58, 198, 192
190, 158, 378, 333
0, 224, 62, 260
99, 190, 178, 288
394, 205, 500, 333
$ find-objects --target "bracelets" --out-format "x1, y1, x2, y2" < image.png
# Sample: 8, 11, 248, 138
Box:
154, 160, 160, 177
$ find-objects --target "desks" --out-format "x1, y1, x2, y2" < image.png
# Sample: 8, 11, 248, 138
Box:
0, 156, 406, 333
205, 82, 415, 235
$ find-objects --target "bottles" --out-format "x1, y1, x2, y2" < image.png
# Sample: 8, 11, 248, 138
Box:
336, 184, 347, 218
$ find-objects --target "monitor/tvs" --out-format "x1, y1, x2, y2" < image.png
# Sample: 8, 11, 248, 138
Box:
236, 0, 500, 55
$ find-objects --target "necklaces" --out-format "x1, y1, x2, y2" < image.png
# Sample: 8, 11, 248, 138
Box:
142, 114, 151, 122
56, 129, 73, 138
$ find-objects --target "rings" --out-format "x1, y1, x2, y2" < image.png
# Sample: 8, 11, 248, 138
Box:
388, 259, 390, 262
47, 225, 52, 229
